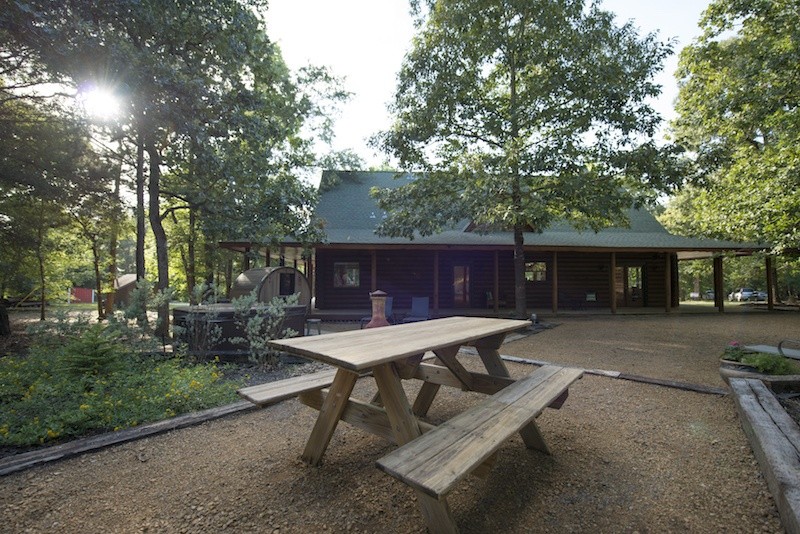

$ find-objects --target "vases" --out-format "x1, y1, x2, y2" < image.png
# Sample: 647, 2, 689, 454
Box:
717, 356, 769, 387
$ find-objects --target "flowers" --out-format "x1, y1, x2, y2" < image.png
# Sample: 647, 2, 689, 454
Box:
722, 340, 758, 362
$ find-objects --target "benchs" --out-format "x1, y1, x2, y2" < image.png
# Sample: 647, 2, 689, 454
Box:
485, 290, 508, 309
237, 364, 583, 496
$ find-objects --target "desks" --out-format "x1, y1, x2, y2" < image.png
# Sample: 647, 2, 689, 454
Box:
269, 316, 549, 488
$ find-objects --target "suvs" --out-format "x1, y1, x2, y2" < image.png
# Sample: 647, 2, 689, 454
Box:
737, 288, 754, 301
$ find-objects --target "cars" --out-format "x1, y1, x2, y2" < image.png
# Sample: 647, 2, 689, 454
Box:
758, 292, 768, 300
706, 291, 714, 295
734, 289, 740, 300
728, 292, 734, 302
754, 291, 757, 296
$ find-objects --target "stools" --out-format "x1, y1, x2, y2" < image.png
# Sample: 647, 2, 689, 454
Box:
305, 318, 321, 337
